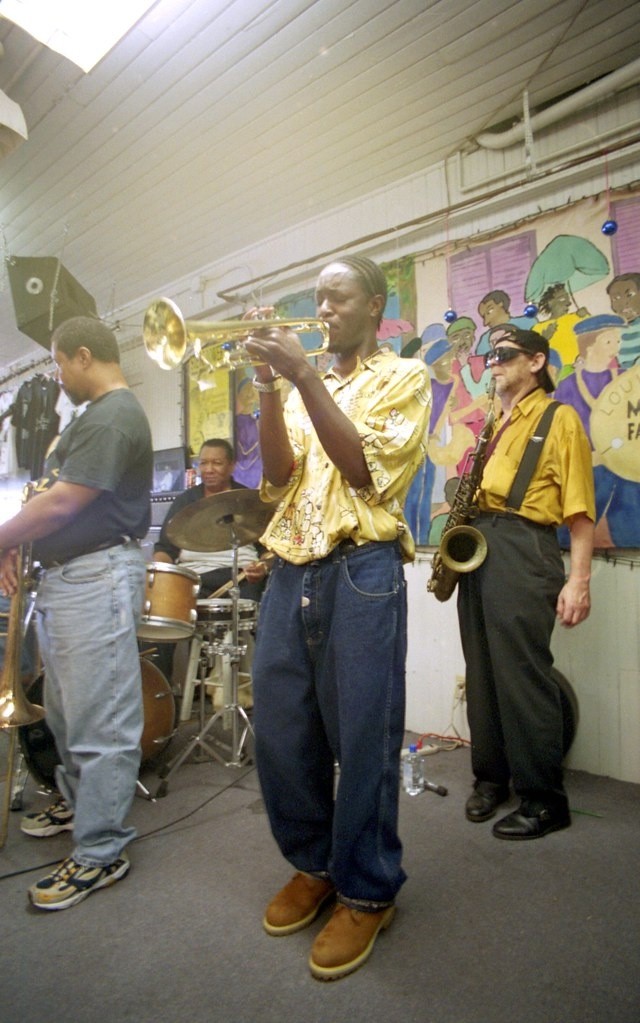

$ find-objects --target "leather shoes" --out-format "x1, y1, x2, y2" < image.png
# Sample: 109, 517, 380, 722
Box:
491, 801, 572, 840
465, 780, 510, 821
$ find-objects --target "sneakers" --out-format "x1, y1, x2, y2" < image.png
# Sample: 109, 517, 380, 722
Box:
28, 850, 130, 909
19, 799, 74, 837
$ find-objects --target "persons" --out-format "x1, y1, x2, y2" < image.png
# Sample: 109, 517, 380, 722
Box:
0, 317, 152, 913
243, 258, 434, 982
154, 440, 276, 711
443, 326, 597, 839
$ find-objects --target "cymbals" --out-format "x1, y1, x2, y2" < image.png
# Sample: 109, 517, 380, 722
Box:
165, 488, 275, 553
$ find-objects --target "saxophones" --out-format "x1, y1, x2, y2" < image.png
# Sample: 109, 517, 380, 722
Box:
424, 376, 499, 602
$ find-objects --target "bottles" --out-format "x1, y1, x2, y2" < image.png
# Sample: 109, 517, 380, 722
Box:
403, 744, 425, 796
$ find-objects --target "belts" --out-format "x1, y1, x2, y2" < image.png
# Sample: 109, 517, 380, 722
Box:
42, 535, 126, 568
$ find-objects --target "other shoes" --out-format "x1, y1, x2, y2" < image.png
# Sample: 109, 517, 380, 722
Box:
308, 902, 394, 982
263, 870, 333, 937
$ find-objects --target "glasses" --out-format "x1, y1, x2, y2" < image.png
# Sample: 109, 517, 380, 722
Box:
483, 347, 533, 369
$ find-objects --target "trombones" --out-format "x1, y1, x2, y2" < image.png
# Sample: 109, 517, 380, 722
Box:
0, 480, 45, 851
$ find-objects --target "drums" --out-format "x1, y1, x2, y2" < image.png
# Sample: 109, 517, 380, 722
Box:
197, 596, 258, 633
18, 656, 179, 791
136, 561, 202, 643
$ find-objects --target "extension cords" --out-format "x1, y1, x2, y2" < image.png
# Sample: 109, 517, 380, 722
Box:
401, 743, 439, 760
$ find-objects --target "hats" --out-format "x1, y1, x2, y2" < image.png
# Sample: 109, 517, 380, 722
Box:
493, 329, 555, 393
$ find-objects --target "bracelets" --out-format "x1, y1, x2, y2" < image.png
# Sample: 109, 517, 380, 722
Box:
252, 373, 283, 393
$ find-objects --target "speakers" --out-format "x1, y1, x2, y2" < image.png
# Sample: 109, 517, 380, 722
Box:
5, 256, 97, 352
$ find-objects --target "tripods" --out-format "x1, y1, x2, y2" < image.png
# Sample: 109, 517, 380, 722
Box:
155, 523, 255, 797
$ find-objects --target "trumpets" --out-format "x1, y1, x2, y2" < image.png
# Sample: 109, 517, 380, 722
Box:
140, 297, 330, 377
